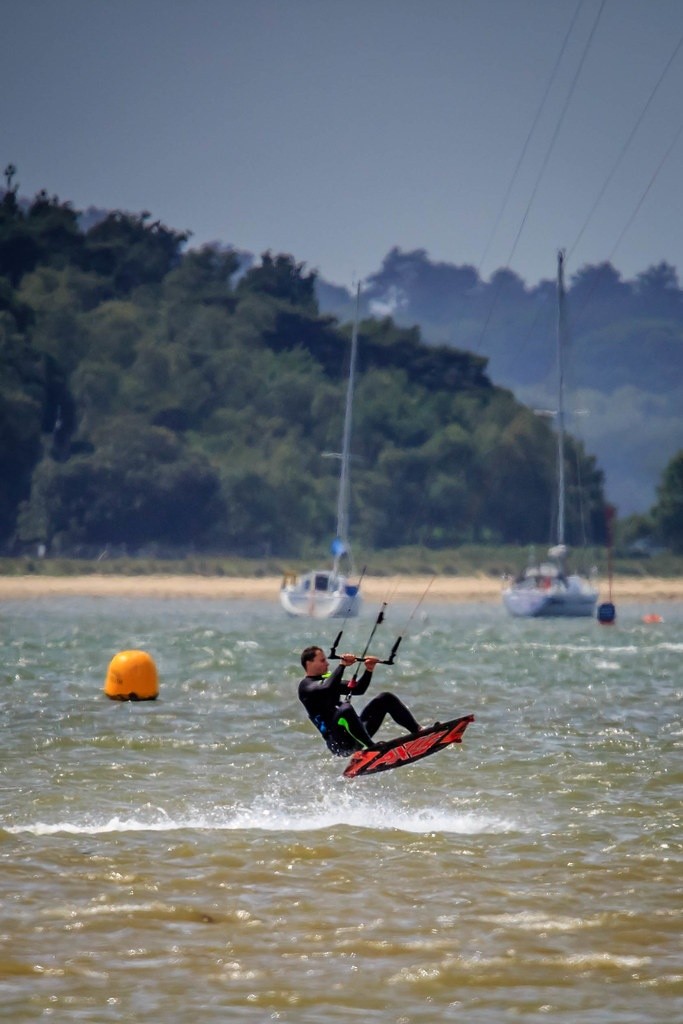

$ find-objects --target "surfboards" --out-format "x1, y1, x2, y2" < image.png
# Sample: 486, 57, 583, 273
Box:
343, 713, 476, 779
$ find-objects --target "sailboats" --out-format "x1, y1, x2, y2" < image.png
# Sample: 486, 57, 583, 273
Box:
279, 280, 362, 620
499, 249, 601, 618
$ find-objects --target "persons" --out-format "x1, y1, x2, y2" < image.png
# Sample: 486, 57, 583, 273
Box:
297, 645, 427, 758
